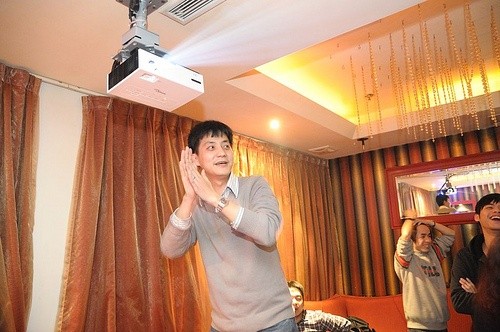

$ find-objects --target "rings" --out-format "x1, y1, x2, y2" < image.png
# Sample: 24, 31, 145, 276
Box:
193, 176, 196, 180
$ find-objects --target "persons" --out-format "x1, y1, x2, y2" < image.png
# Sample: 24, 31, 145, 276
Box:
394, 210, 456, 332
160, 120, 297, 332
450, 193, 500, 332
436, 194, 456, 214
287, 280, 351, 332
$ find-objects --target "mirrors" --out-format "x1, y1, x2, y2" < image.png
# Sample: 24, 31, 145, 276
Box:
385, 150, 500, 229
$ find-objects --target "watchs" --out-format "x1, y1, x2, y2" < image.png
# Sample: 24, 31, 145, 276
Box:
433, 220, 436, 226
214, 198, 229, 213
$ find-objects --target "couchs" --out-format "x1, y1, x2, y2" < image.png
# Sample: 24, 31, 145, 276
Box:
304, 293, 472, 332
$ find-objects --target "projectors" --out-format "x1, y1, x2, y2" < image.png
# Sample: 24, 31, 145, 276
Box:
107, 47, 204, 112
